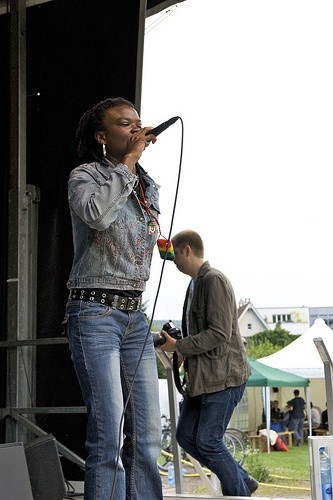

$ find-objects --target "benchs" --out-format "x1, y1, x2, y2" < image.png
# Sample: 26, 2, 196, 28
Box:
238, 429, 252, 439
314, 429, 328, 435
301, 426, 317, 443
248, 430, 296, 450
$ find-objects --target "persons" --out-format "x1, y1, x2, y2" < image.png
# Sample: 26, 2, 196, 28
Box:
67, 98, 163, 500
262, 389, 329, 446
157, 231, 259, 497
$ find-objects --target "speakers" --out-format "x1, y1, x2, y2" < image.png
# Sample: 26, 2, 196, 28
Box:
25, 434, 67, 500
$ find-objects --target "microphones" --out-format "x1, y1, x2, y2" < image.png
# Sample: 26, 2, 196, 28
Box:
144, 116, 179, 147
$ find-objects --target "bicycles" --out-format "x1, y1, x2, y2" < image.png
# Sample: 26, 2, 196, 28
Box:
156, 412, 254, 475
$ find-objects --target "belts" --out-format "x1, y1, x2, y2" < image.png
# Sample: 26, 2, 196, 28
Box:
68, 289, 141, 311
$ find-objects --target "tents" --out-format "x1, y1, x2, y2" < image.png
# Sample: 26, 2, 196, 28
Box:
257, 317, 332, 436
173, 359, 311, 455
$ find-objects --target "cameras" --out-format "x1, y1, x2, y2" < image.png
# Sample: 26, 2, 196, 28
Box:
152, 321, 182, 346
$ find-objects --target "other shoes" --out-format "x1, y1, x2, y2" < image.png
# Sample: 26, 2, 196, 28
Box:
296, 439, 301, 446
247, 474, 259, 494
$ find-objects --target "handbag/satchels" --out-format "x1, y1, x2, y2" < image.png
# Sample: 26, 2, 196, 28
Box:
273, 437, 288, 451
